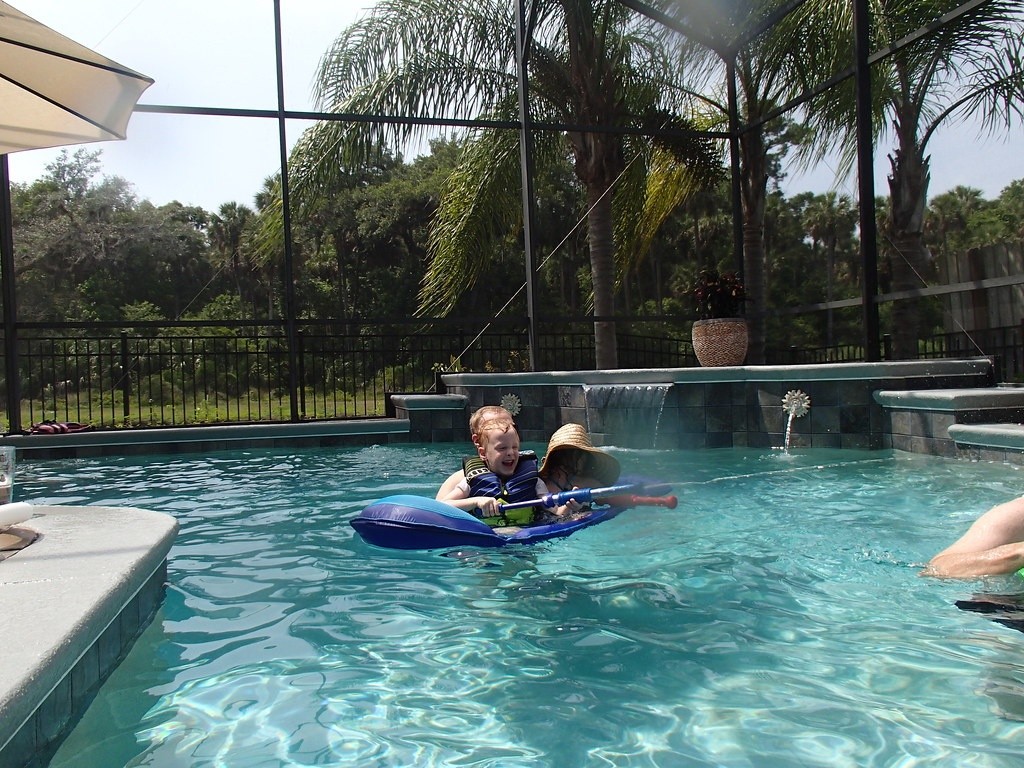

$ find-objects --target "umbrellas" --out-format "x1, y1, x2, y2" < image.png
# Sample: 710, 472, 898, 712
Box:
0, 0, 154, 156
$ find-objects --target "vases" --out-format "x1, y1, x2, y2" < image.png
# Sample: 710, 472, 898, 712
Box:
691, 316, 749, 366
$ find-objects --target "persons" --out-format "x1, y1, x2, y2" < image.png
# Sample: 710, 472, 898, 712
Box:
930, 498, 1024, 577
538, 423, 621, 517
435, 406, 583, 530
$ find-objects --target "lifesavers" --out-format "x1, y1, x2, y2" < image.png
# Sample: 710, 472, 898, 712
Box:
348, 472, 675, 552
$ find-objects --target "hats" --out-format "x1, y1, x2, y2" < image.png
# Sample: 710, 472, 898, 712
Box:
539, 423, 620, 494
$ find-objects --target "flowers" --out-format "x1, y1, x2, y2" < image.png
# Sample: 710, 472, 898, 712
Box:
692, 270, 744, 319
